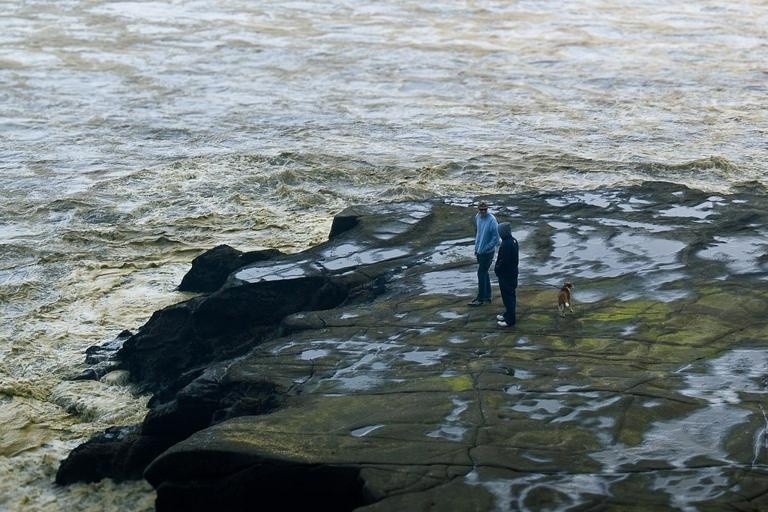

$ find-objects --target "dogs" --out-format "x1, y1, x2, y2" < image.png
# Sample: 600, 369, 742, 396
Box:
558, 282, 574, 318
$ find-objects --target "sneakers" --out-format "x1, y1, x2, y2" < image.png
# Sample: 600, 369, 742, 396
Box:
496, 315, 504, 320
468, 297, 491, 306
498, 321, 509, 327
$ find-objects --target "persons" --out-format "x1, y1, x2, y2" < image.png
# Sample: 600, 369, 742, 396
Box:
465, 202, 501, 308
494, 220, 519, 329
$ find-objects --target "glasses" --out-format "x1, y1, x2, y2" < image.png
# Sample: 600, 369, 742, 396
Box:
479, 208, 487, 210
